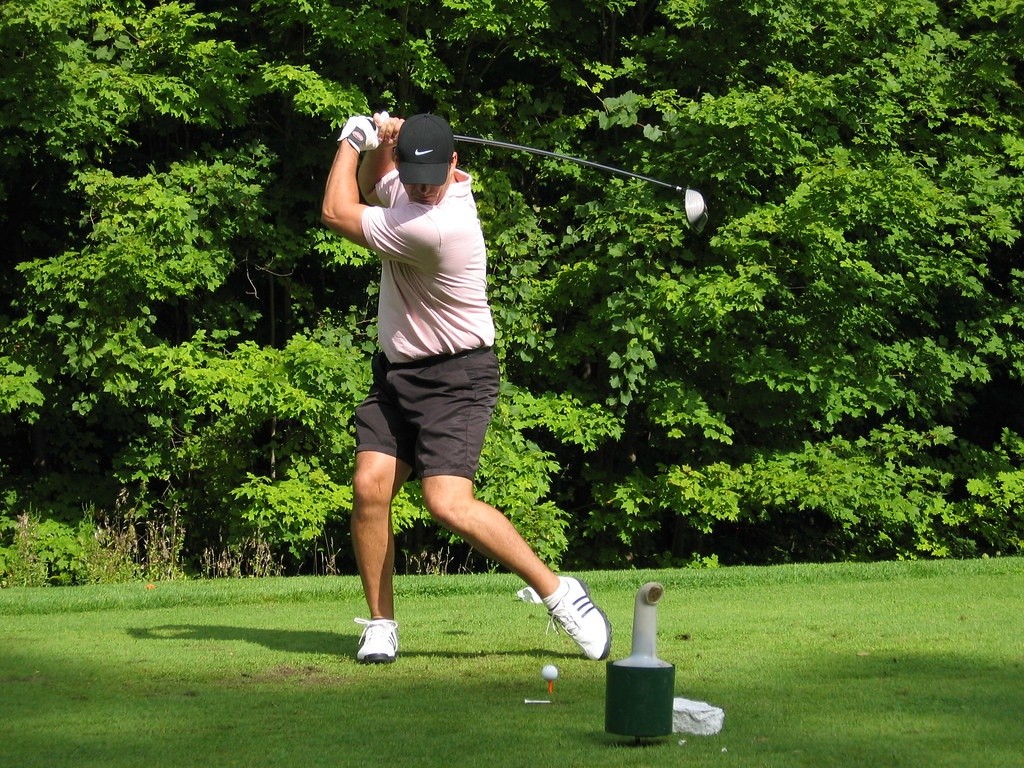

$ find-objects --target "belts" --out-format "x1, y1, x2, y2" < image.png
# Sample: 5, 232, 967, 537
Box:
391, 346, 492, 370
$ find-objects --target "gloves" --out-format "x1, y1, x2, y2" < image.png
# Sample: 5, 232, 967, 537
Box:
336, 114, 383, 155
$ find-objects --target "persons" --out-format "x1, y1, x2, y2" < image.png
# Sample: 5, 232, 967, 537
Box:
321, 110, 612, 663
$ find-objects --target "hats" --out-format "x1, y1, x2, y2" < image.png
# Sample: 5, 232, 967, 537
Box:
395, 113, 453, 186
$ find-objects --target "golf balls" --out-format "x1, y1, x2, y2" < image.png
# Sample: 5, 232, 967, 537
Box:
541, 665, 559, 680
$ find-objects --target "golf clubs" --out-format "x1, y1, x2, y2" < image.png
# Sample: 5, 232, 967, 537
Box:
452, 132, 709, 237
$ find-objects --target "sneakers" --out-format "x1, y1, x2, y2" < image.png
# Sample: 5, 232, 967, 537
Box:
545, 576, 612, 660
353, 617, 398, 662
